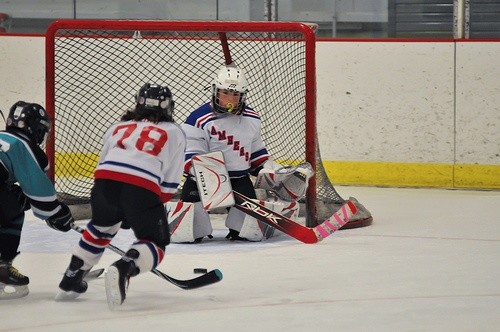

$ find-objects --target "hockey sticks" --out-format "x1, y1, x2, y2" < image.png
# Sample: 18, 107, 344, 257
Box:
70, 223, 223, 291
182, 172, 359, 244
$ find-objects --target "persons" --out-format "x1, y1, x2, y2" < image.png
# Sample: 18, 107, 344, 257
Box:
59, 83, 186, 308
180, 67, 284, 242
0, 101, 75, 299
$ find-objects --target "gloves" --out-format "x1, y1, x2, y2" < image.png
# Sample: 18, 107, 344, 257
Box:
45, 202, 75, 232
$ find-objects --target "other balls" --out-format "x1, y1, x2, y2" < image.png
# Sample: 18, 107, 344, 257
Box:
193, 268, 208, 274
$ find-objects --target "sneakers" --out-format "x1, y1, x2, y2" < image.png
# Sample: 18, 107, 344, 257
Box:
104, 248, 141, 312
0, 252, 30, 300
56, 255, 88, 300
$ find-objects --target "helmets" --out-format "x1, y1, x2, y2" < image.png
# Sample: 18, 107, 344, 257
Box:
5, 101, 51, 150
135, 83, 174, 121
211, 67, 248, 115
